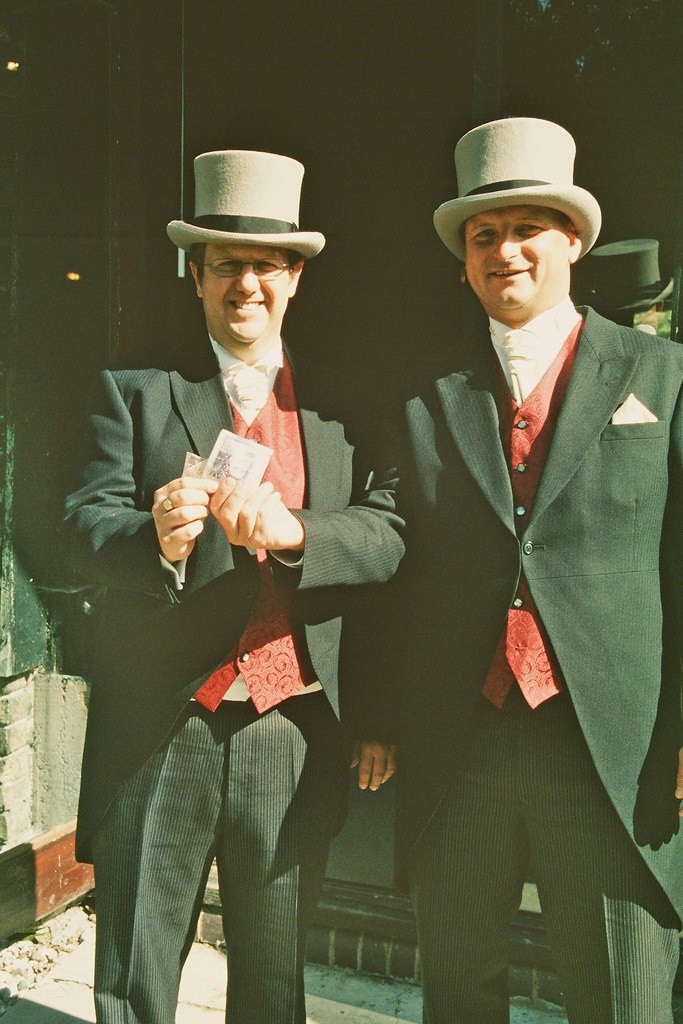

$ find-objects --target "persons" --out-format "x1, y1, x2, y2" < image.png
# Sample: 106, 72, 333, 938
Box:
57, 146, 401, 1024
203, 118, 683, 1023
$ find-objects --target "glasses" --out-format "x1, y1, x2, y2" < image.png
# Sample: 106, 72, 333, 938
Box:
193, 257, 291, 278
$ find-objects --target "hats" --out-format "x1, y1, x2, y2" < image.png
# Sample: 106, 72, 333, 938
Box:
578, 235, 673, 313
433, 117, 601, 261
165, 149, 325, 261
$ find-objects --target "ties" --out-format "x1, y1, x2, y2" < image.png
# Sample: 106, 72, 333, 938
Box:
223, 369, 267, 703
504, 329, 533, 408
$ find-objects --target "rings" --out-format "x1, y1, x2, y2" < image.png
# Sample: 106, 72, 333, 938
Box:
161, 496, 172, 510
